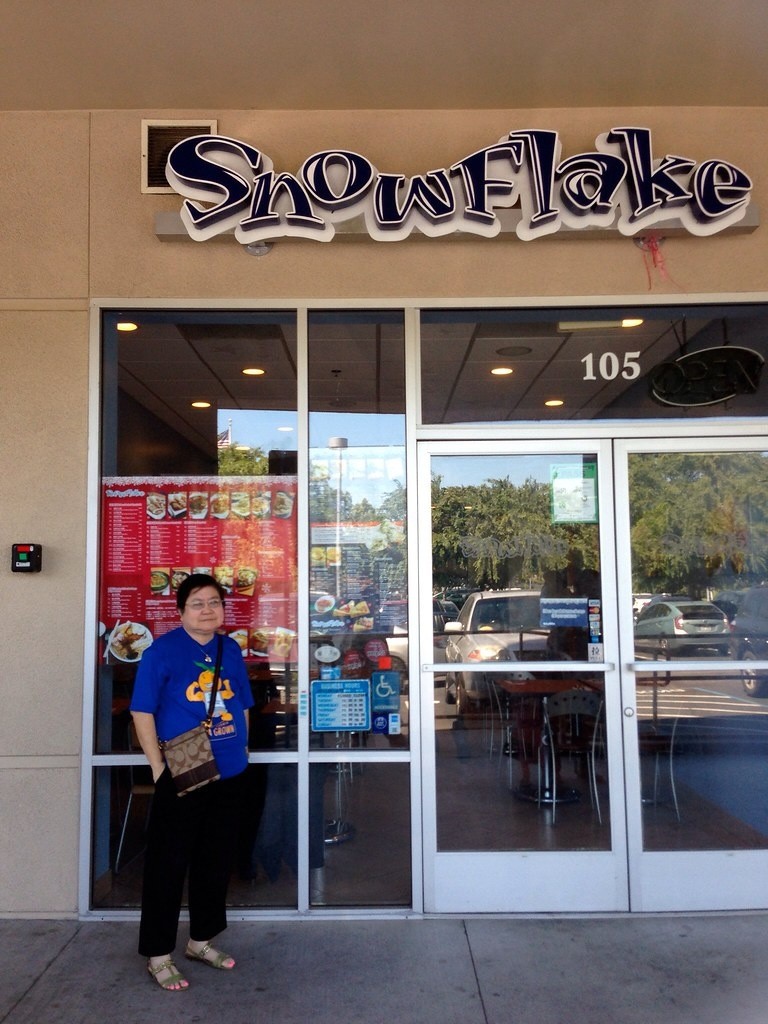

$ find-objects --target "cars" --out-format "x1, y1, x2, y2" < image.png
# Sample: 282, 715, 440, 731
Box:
640, 595, 703, 611
629, 600, 730, 657
444, 589, 553, 715
307, 588, 460, 691
712, 589, 745, 617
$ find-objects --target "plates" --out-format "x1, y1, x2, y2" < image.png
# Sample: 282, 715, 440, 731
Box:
189, 494, 208, 519
314, 596, 335, 612
109, 622, 153, 662
210, 494, 229, 519
252, 498, 269, 515
250, 649, 268, 656
166, 494, 187, 515
228, 631, 247, 657
231, 492, 250, 517
146, 496, 165, 519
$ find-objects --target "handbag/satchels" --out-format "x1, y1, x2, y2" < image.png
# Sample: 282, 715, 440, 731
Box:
164, 724, 220, 798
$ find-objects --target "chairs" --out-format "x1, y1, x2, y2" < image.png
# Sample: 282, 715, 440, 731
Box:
640, 690, 688, 822
541, 688, 604, 825
113, 713, 155, 873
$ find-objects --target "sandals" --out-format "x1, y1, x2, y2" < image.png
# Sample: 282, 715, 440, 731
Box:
185, 941, 235, 970
147, 959, 190, 991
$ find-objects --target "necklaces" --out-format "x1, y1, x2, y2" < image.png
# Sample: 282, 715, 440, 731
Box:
183, 632, 214, 662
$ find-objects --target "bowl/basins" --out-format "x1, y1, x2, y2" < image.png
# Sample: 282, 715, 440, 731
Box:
151, 571, 169, 590
172, 572, 190, 589
238, 569, 256, 588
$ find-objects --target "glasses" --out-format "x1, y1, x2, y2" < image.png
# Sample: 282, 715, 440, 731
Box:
185, 600, 225, 610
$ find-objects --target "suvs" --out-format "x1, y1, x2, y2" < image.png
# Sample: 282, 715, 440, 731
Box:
729, 581, 768, 698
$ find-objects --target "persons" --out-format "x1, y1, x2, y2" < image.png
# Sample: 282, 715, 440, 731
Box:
128, 573, 253, 991
540, 549, 599, 658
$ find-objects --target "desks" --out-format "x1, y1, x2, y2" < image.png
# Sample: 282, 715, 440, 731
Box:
494, 678, 685, 804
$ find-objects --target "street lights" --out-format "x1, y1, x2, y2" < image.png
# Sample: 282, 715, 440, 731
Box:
326, 438, 350, 603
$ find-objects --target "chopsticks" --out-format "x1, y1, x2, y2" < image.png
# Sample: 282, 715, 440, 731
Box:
103, 619, 120, 657
